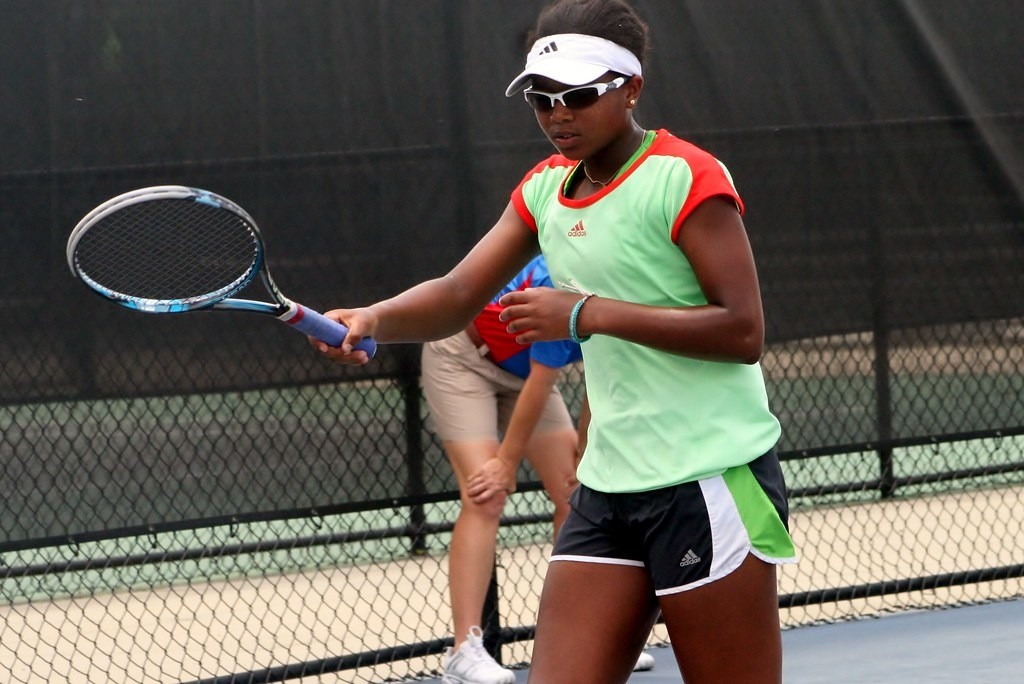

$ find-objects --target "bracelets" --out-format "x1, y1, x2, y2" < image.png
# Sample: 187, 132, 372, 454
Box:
557, 276, 596, 344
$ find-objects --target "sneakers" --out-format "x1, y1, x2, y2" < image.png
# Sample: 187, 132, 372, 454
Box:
633, 652, 655, 671
442, 626, 516, 684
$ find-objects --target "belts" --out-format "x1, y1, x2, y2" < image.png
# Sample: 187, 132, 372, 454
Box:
465, 320, 507, 371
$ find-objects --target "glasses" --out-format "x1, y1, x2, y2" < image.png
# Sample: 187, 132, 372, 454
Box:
524, 77, 628, 112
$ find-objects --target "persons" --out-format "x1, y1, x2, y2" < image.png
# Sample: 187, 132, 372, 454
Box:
310, 1, 801, 684
421, 249, 658, 683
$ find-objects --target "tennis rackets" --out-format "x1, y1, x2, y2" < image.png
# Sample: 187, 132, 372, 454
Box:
65, 184, 378, 361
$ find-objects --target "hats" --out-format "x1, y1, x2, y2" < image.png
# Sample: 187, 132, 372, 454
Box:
506, 34, 641, 98
